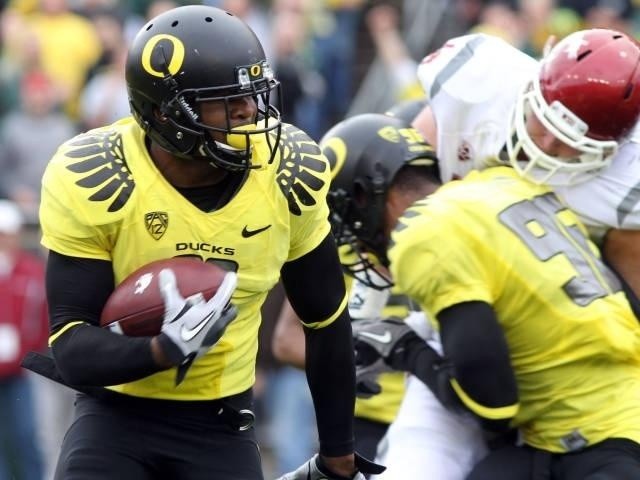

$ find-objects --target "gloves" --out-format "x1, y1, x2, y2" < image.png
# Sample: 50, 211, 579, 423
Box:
353, 316, 422, 397
278, 452, 385, 480
158, 268, 241, 383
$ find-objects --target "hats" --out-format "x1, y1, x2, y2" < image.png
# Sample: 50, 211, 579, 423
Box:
124, 4, 282, 171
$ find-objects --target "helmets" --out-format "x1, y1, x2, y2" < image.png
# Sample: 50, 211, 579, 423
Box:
318, 112, 440, 291
507, 28, 640, 186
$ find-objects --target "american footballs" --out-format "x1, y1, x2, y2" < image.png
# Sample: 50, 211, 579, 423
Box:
100, 257, 226, 338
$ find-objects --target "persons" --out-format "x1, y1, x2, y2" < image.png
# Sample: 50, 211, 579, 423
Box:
214, 2, 639, 149
360, 23, 639, 480
1, 1, 173, 480
40, 6, 388, 479
319, 111, 638, 479
254, 255, 412, 480
